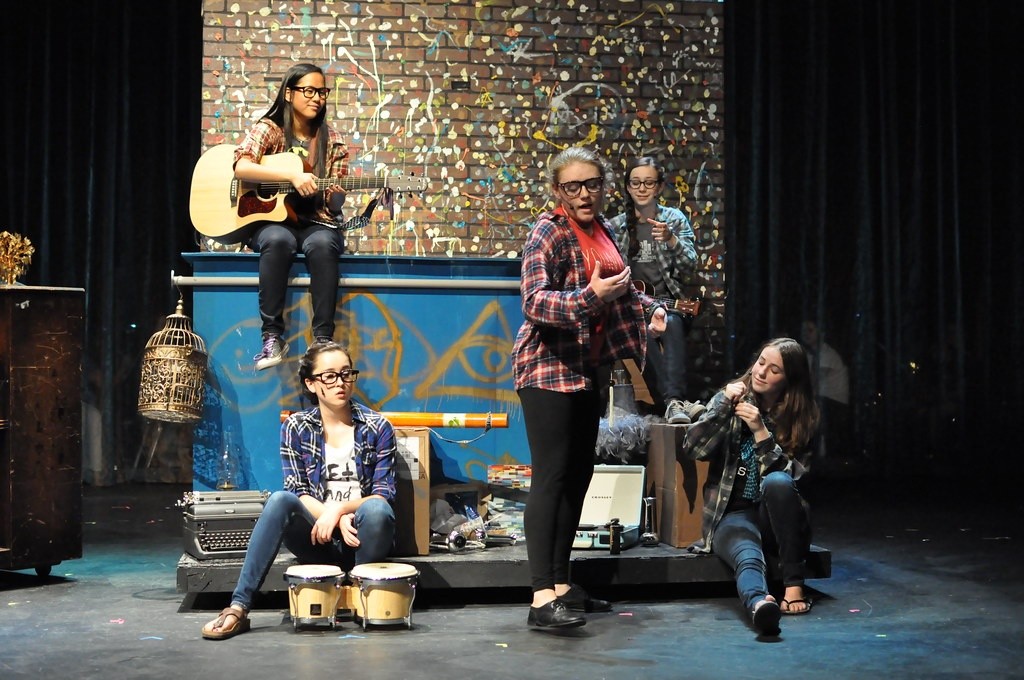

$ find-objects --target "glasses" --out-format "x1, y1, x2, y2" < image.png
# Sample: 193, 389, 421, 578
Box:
629, 179, 659, 189
288, 85, 330, 100
311, 369, 360, 385
553, 175, 604, 198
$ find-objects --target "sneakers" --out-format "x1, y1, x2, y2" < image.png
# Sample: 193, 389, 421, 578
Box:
253, 331, 290, 371
683, 400, 708, 419
312, 335, 333, 346
664, 400, 691, 424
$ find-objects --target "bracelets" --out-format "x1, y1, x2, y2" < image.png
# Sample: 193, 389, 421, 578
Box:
665, 227, 675, 242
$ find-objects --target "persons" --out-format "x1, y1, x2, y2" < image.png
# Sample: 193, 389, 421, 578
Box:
795, 319, 852, 406
199, 333, 400, 641
597, 157, 708, 425
510, 145, 668, 629
232, 63, 348, 370
682, 338, 821, 641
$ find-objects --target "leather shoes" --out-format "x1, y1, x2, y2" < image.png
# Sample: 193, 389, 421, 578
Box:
527, 597, 587, 630
552, 584, 611, 613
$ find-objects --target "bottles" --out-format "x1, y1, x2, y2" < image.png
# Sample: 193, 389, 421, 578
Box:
640, 497, 660, 547
609, 519, 621, 555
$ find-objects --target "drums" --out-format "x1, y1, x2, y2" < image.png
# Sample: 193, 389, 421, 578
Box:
348, 562, 421, 632
284, 564, 346, 632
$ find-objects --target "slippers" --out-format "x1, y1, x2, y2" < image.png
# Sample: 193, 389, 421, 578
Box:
201, 607, 251, 640
751, 601, 781, 636
780, 598, 808, 614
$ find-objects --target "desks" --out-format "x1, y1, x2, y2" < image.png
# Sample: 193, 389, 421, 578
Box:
0, 284, 85, 577
644, 422, 711, 548
394, 426, 431, 556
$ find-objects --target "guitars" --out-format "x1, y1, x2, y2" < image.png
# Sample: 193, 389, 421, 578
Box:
631, 278, 705, 318
188, 143, 430, 246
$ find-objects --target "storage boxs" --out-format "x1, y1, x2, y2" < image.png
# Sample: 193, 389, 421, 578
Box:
572, 463, 645, 548
